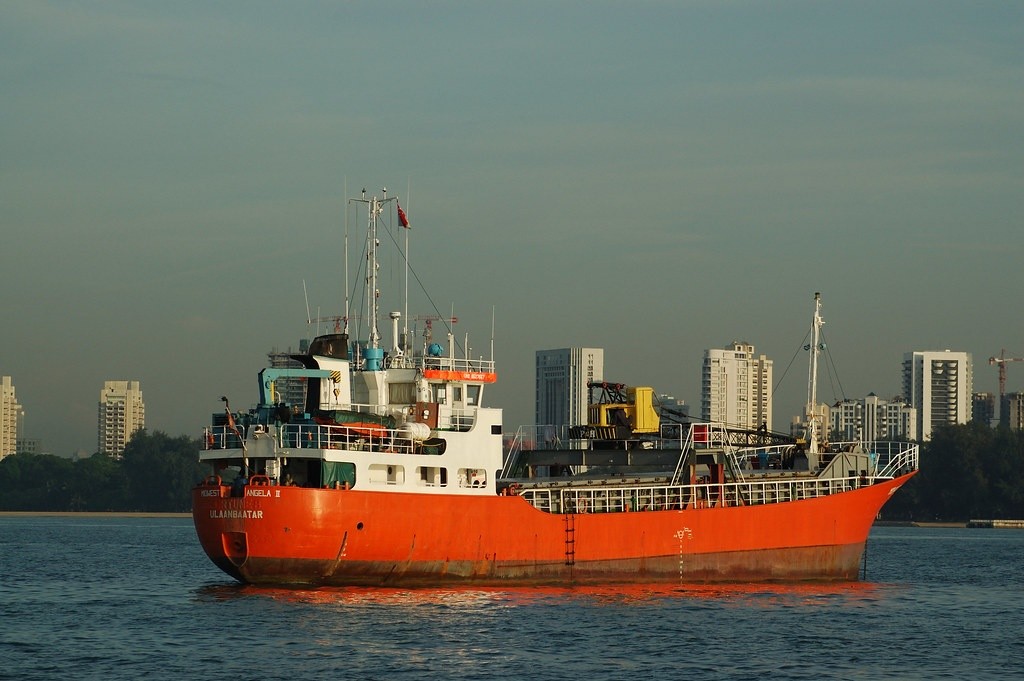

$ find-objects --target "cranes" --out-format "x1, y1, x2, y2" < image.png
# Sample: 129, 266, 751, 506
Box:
986, 340, 1024, 394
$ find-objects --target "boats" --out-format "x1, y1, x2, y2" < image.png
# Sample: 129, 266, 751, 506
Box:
191, 175, 921, 589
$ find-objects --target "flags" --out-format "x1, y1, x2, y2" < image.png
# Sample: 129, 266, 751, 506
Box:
398, 206, 410, 229
